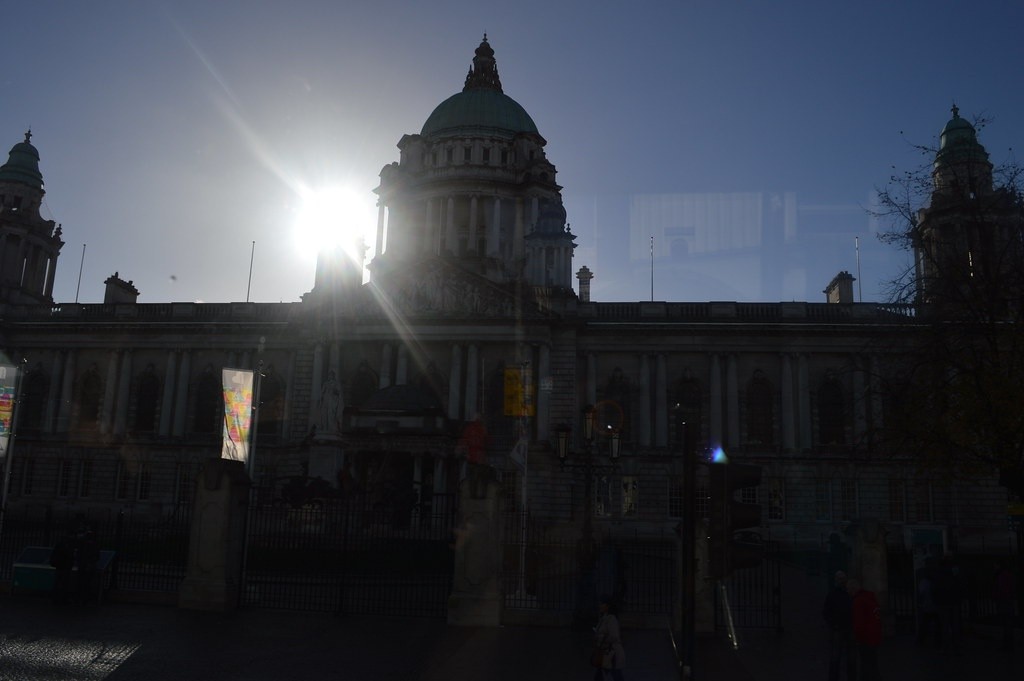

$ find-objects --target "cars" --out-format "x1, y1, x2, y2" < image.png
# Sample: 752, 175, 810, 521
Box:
733, 526, 780, 556
255, 474, 332, 511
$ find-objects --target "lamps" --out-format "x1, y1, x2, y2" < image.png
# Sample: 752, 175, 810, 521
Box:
553, 423, 572, 460
607, 425, 624, 461
581, 404, 598, 442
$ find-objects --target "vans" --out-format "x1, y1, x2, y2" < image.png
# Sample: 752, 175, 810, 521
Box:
883, 523, 953, 556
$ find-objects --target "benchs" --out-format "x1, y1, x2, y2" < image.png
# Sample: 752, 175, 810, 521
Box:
9, 546, 116, 605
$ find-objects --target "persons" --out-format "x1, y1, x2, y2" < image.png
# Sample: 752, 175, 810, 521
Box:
453, 412, 488, 497
916, 555, 963, 653
593, 593, 626, 681
824, 570, 882, 681
992, 560, 1014, 655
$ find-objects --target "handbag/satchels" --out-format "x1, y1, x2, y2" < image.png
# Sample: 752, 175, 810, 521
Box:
591, 634, 609, 668
